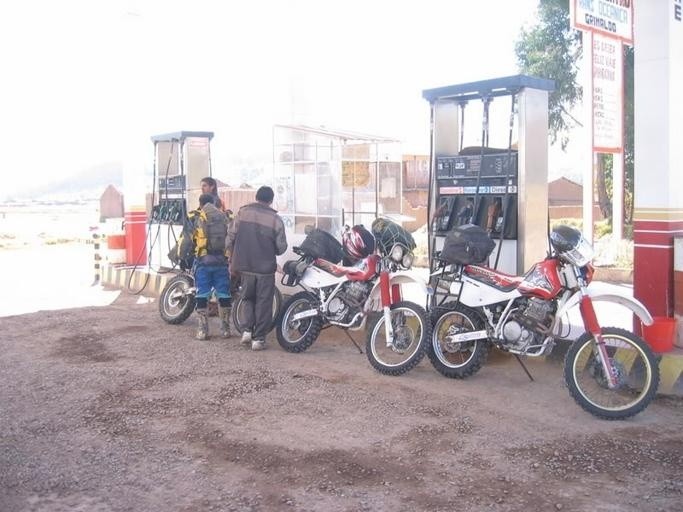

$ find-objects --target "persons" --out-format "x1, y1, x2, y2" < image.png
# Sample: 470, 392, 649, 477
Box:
228, 186, 287, 350
197, 178, 225, 211
177, 194, 235, 341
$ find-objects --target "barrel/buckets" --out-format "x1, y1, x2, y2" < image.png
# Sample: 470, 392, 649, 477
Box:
641, 316, 678, 354
109, 249, 127, 264
107, 234, 125, 249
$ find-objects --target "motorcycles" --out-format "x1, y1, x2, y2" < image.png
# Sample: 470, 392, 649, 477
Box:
271, 217, 432, 376
423, 223, 660, 422
156, 267, 281, 341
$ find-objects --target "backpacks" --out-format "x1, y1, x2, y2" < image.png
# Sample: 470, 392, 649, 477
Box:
199, 214, 226, 253
298, 228, 341, 265
440, 222, 495, 267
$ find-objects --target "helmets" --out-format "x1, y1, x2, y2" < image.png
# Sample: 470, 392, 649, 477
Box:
548, 223, 584, 257
340, 223, 376, 265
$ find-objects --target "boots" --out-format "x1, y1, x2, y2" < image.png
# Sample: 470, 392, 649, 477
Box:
218, 306, 232, 339
194, 307, 213, 340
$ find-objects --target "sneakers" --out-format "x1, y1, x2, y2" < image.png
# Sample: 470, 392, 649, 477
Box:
250, 339, 268, 351
239, 331, 252, 347
